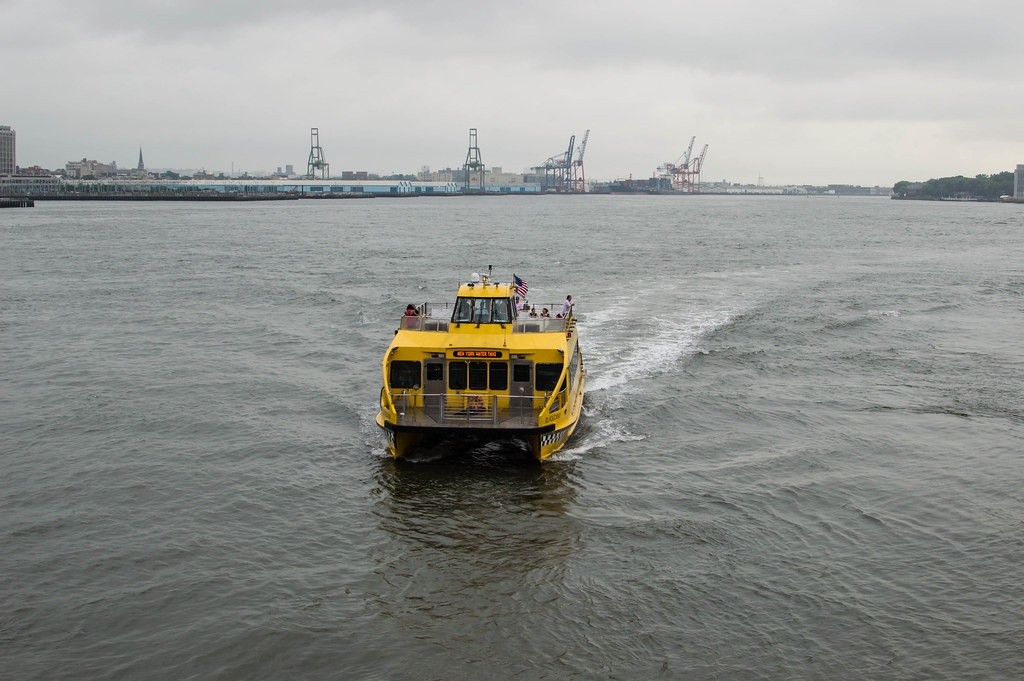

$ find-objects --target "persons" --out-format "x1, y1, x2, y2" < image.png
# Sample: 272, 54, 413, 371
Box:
516, 296, 523, 310
540, 308, 550, 317
404, 304, 420, 329
562, 295, 575, 316
530, 308, 537, 318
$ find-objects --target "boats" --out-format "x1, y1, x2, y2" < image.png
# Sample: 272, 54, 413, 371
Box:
376, 263, 589, 465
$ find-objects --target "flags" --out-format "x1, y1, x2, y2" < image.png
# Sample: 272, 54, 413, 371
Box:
513, 273, 529, 298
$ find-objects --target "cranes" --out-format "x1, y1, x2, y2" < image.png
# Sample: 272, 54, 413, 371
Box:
660, 135, 710, 189
307, 127, 330, 180
529, 129, 588, 193
462, 128, 485, 190
